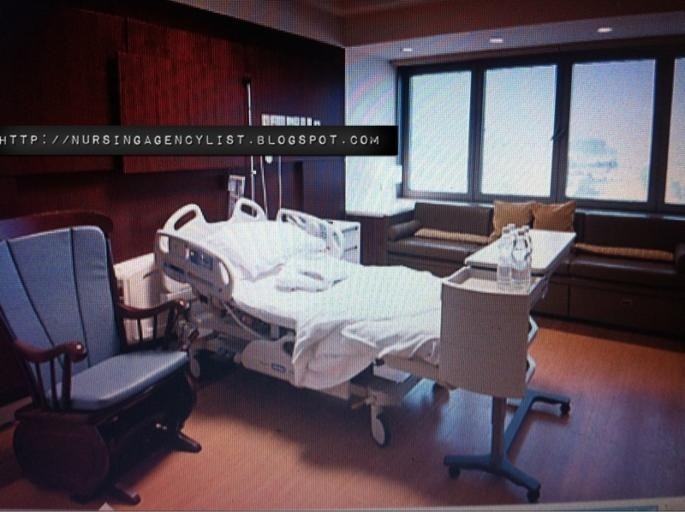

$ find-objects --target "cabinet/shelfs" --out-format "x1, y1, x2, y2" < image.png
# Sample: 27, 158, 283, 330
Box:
438, 226, 574, 502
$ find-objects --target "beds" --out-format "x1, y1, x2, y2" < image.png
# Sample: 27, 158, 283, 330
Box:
152, 196, 438, 450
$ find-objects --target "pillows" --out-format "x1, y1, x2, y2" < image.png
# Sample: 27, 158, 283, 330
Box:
530, 199, 576, 234
207, 219, 326, 281
487, 198, 537, 246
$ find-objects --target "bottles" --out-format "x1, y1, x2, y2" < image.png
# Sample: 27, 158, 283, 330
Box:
496, 222, 533, 290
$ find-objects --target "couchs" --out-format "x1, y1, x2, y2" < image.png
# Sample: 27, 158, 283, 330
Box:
385, 196, 685, 350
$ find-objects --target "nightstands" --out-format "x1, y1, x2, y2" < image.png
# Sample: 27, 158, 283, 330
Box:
324, 218, 362, 265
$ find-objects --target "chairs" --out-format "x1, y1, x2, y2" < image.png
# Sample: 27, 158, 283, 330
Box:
0, 210, 201, 506
